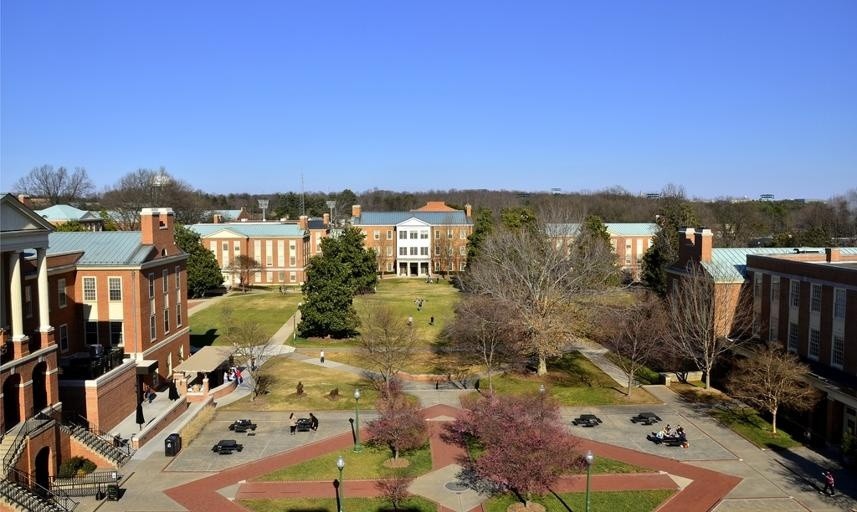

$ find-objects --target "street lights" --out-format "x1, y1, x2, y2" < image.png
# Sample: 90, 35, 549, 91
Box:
353, 389, 362, 454
539, 384, 545, 449
337, 456, 344, 512
585, 450, 593, 512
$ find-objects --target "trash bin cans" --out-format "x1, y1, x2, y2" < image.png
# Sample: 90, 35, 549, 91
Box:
165, 433, 181, 456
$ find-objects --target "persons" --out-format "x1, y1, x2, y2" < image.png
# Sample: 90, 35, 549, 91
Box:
144, 385, 148, 398
289, 413, 297, 435
417, 299, 423, 311
309, 413, 318, 431
415, 299, 421, 312
427, 275, 430, 284
235, 368, 241, 385
655, 423, 686, 448
222, 368, 230, 383
145, 384, 151, 403
429, 314, 434, 326
319, 349, 324, 363
407, 314, 412, 326
